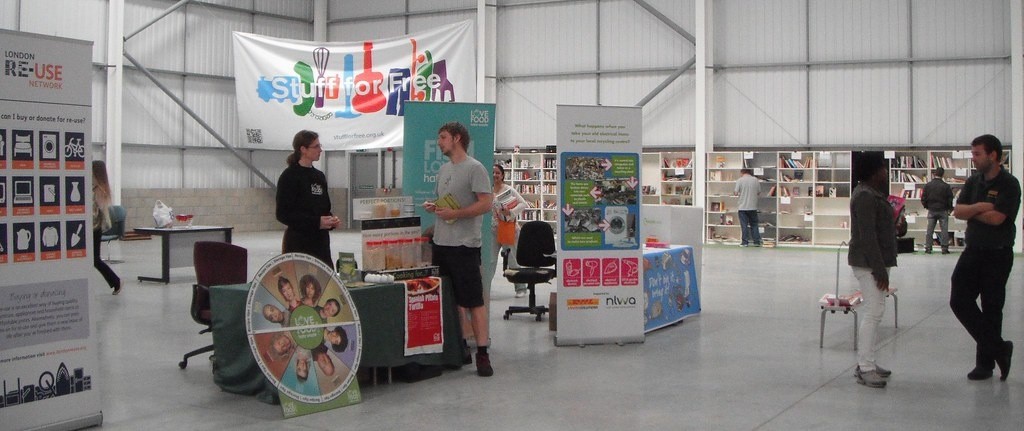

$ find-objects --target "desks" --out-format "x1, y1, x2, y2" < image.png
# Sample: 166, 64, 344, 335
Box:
209, 275, 472, 403
542, 243, 701, 333
134, 225, 234, 284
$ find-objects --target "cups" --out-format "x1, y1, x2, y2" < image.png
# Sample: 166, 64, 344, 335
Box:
390, 203, 401, 218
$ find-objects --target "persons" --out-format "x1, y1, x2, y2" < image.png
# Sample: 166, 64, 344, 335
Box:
921, 168, 954, 254
949, 134, 1022, 381
92, 160, 125, 295
734, 170, 762, 247
489, 164, 527, 297
422, 122, 493, 376
848, 154, 898, 388
276, 130, 343, 270
565, 157, 636, 232
263, 274, 348, 382
891, 156, 927, 199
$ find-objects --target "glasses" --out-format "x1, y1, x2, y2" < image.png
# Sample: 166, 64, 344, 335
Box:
307, 144, 322, 149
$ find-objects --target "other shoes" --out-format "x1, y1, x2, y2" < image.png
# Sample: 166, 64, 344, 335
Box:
446, 346, 473, 372
112, 279, 124, 295
516, 289, 528, 298
967, 365, 994, 381
475, 353, 494, 377
942, 249, 950, 254
996, 340, 1014, 382
754, 244, 760, 247
925, 250, 931, 254
740, 244, 748, 247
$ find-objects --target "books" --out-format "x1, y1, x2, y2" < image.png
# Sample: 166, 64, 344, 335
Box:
641, 157, 692, 206
495, 188, 517, 222
543, 158, 556, 194
521, 199, 541, 221
779, 155, 813, 196
779, 206, 812, 242
744, 160, 778, 197
819, 293, 860, 307
709, 155, 736, 242
757, 209, 776, 248
543, 199, 557, 221
931, 152, 966, 183
815, 160, 836, 198
495, 158, 541, 195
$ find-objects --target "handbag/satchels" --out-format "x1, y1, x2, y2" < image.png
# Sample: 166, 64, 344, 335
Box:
152, 199, 175, 228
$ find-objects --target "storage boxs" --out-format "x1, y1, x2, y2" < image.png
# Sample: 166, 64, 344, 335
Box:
549, 291, 557, 330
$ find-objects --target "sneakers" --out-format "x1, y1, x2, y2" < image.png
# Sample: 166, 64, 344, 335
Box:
856, 369, 887, 389
853, 363, 892, 378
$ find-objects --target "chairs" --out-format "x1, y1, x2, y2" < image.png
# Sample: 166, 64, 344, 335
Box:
99, 205, 126, 264
500, 221, 556, 321
177, 241, 249, 369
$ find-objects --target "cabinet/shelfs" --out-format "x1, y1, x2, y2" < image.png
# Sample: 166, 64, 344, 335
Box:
928, 150, 970, 250
887, 151, 931, 250
641, 151, 697, 206
706, 150, 778, 247
776, 151, 854, 248
971, 149, 1013, 176
493, 151, 557, 222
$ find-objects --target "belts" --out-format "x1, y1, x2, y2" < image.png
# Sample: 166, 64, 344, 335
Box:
966, 243, 1011, 250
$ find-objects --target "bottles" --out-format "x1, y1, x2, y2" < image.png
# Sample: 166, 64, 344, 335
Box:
336, 252, 357, 274
364, 236, 432, 272
